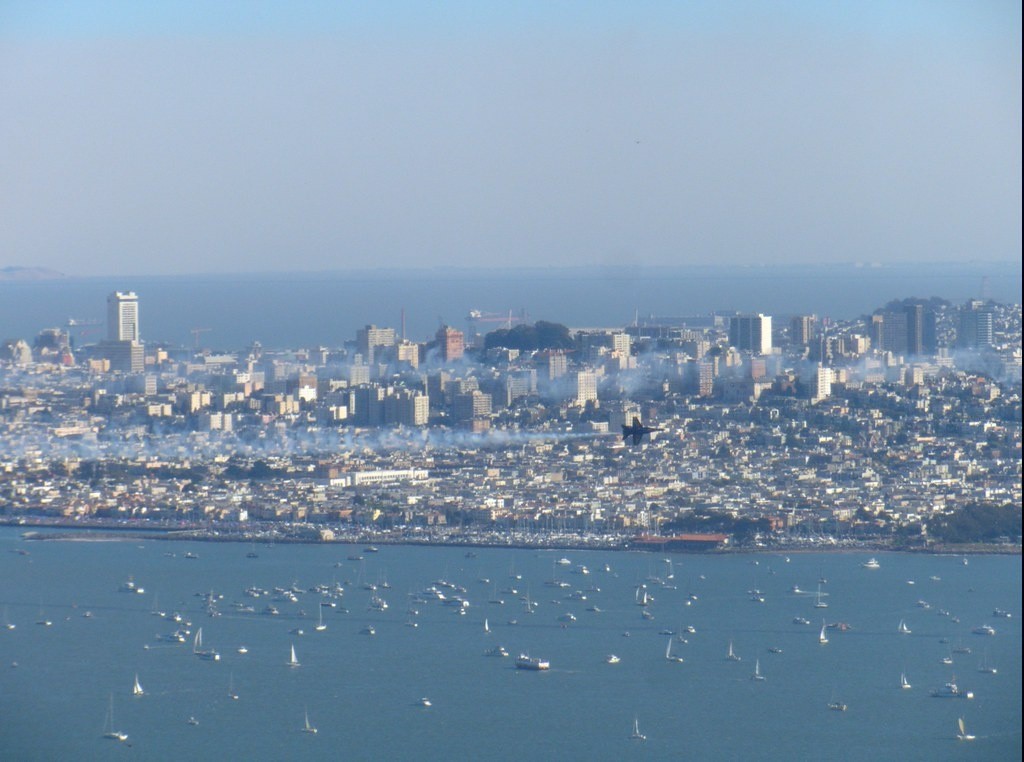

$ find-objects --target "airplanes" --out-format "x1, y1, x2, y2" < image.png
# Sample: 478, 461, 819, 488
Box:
620, 416, 664, 446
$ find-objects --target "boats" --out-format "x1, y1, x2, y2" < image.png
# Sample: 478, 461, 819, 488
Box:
0, 549, 1011, 740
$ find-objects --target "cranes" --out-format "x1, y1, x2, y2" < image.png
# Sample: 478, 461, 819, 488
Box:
463, 308, 531, 331
